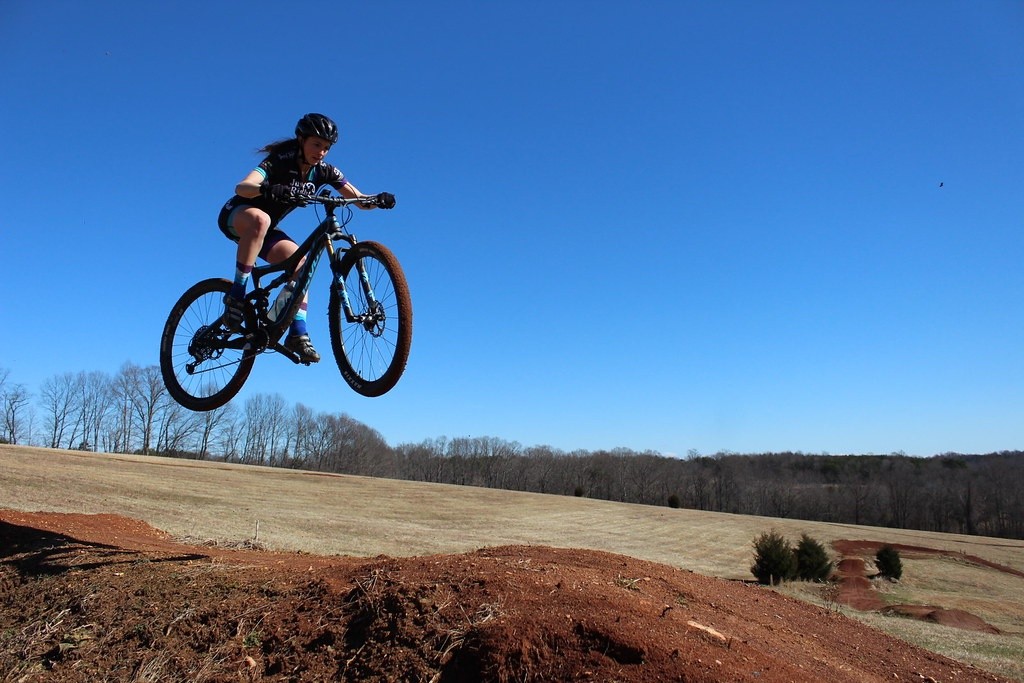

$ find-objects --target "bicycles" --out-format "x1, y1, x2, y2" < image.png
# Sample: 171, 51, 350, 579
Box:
160, 182, 412, 412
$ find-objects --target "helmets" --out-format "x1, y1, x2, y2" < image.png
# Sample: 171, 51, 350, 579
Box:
295, 111, 337, 143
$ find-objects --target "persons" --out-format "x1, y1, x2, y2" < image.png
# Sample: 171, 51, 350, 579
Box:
218, 113, 395, 364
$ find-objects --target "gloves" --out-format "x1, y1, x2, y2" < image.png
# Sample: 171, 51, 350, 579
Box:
259, 184, 290, 201
376, 192, 395, 209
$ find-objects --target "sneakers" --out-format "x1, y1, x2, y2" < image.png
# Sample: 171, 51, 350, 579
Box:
222, 295, 252, 312
285, 334, 320, 362
